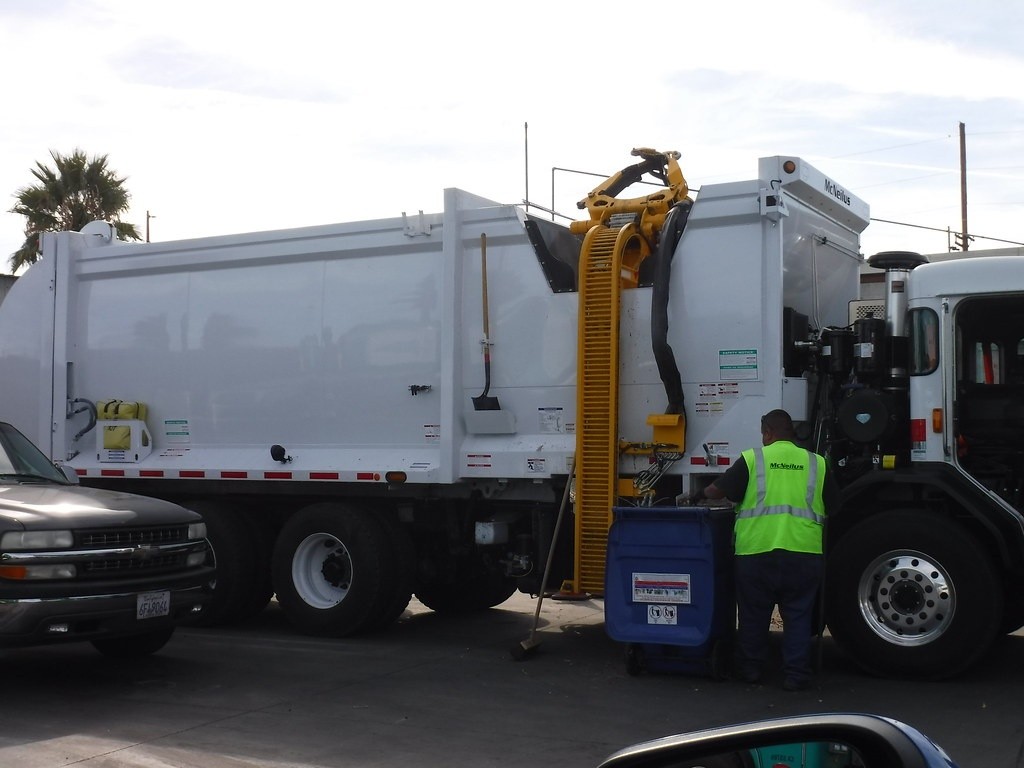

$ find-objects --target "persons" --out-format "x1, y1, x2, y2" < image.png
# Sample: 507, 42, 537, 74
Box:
704, 409, 830, 693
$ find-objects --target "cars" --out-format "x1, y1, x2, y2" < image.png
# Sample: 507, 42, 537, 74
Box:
0, 420, 217, 661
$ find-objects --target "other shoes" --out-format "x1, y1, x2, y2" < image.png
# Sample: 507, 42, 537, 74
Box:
745, 675, 757, 683
785, 682, 810, 692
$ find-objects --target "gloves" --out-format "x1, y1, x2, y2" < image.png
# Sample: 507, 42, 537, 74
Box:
691, 489, 707, 504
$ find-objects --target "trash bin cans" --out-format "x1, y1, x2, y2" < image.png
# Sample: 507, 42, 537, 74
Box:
604, 503, 737, 681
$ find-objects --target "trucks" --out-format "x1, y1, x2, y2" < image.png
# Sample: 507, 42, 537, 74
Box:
0, 155, 1024, 670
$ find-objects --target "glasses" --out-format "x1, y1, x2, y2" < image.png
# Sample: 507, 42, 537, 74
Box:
761, 416, 773, 430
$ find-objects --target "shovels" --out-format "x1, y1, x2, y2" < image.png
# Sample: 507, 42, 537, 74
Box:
471, 232, 501, 411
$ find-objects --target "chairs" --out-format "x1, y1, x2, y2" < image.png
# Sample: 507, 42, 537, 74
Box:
958, 398, 1024, 490
